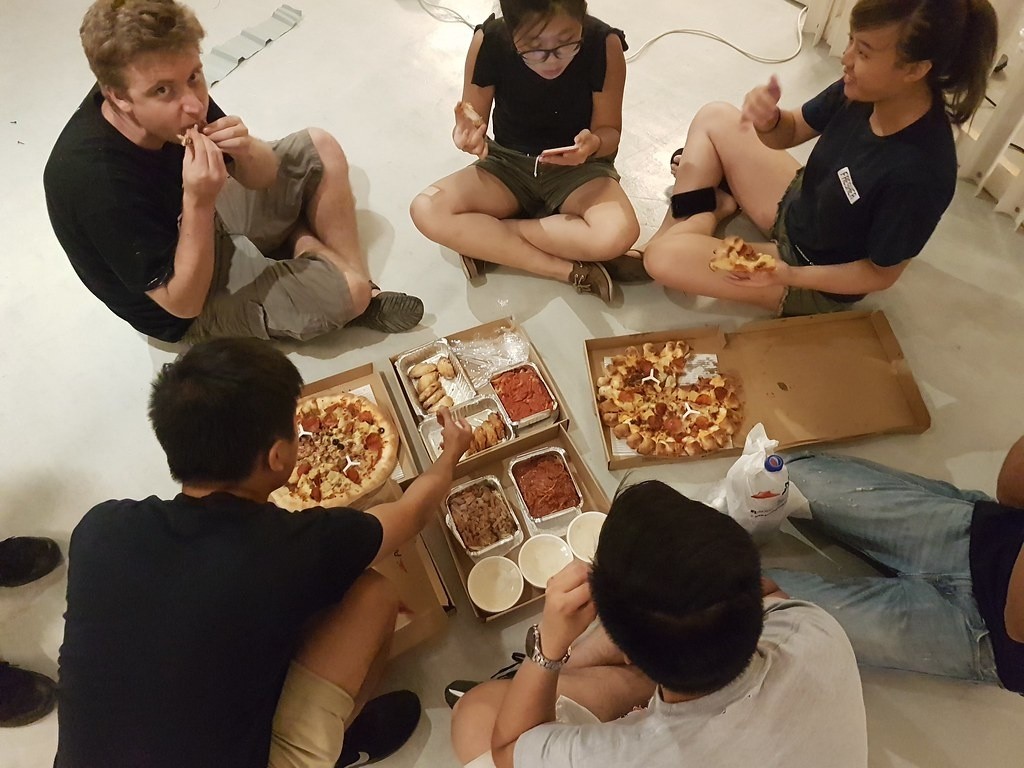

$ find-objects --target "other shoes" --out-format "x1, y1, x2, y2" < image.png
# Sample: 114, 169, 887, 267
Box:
0, 661, 56, 727
0, 537, 61, 587
445, 653, 525, 708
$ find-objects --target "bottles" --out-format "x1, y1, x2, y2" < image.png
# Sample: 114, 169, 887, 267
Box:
746, 454, 789, 536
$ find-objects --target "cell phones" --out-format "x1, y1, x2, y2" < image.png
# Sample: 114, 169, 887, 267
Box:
670, 187, 716, 218
539, 145, 579, 157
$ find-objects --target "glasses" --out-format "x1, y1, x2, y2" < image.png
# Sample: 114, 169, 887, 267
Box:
515, 26, 585, 62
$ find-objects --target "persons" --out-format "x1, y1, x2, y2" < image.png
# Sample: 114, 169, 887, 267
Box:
444, 468, 867, 768
642, 0, 1001, 315
408, 0, 644, 304
0, 535, 64, 729
42, 0, 424, 342
759, 435, 1024, 699
51, 335, 475, 768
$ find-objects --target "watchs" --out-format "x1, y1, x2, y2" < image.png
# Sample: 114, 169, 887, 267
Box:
525, 623, 573, 671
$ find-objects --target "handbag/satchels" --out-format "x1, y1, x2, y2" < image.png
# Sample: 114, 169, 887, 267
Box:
714, 422, 834, 563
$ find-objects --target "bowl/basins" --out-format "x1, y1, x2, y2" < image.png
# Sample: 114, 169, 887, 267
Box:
397, 337, 476, 419
566, 511, 608, 564
508, 446, 584, 523
418, 394, 515, 465
489, 361, 558, 426
467, 556, 524, 612
444, 475, 521, 555
518, 533, 574, 589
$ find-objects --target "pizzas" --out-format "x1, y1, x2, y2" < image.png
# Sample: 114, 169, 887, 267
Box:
596, 340, 742, 457
709, 235, 778, 273
269, 393, 398, 512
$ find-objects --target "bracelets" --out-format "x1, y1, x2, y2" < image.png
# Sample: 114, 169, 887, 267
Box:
754, 106, 781, 134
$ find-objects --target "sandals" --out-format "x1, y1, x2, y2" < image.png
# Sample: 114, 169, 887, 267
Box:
344, 280, 425, 334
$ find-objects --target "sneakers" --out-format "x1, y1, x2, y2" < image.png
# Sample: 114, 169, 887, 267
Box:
335, 690, 421, 768
570, 260, 613, 303
460, 251, 484, 280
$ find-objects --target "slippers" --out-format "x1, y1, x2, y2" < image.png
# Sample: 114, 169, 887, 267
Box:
671, 148, 733, 198
601, 249, 655, 282
